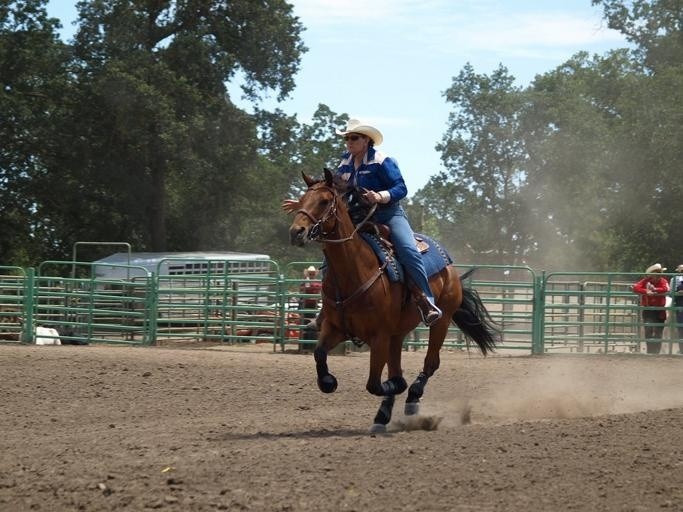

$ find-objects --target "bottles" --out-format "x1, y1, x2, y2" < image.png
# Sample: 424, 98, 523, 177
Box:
303, 277, 312, 287
646, 281, 653, 294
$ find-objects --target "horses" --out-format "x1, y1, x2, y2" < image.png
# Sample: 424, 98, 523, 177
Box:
288, 167, 515, 440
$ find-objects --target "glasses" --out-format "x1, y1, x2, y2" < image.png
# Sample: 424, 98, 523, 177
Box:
343, 135, 362, 141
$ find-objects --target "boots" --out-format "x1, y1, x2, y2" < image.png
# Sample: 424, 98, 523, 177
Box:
307, 307, 323, 331
415, 292, 439, 323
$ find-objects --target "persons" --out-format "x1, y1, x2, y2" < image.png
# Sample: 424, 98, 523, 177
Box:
281, 117, 442, 331
633, 263, 669, 354
669, 264, 683, 355
297, 265, 322, 349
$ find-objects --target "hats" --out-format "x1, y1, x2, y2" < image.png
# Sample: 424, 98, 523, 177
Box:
675, 265, 683, 272
304, 266, 319, 278
646, 263, 667, 273
336, 119, 383, 145
